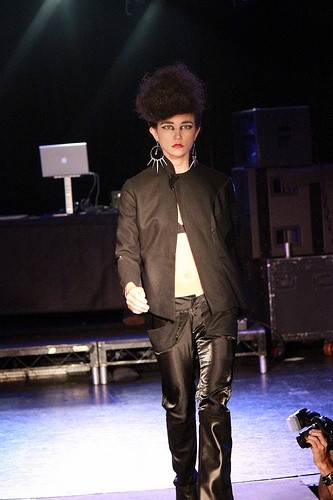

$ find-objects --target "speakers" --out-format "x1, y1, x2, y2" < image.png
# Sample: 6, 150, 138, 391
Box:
231, 105, 333, 260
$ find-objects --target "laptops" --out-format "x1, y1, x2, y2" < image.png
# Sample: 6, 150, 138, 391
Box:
112, 191, 120, 209
38, 142, 95, 176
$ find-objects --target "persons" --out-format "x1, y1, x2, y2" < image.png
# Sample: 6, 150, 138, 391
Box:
306, 429, 333, 500
321, 471, 333, 487
116, 62, 254, 500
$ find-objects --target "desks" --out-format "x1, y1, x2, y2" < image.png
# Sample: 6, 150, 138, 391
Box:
0, 211, 128, 317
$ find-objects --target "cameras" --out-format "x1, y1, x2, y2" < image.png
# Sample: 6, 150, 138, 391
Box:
286, 408, 333, 450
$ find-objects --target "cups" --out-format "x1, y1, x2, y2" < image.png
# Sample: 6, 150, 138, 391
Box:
284, 242, 293, 258
